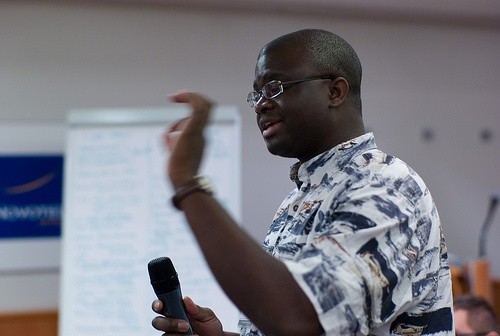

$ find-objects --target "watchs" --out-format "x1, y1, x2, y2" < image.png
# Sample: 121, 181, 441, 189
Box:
172, 176, 215, 210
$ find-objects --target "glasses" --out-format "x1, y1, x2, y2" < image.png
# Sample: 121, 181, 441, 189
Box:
247, 74, 336, 108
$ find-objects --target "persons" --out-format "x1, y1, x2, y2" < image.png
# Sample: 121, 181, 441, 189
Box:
152, 28, 456, 336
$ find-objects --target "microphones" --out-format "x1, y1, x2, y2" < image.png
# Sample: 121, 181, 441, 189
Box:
148, 257, 193, 335
479, 196, 498, 257
